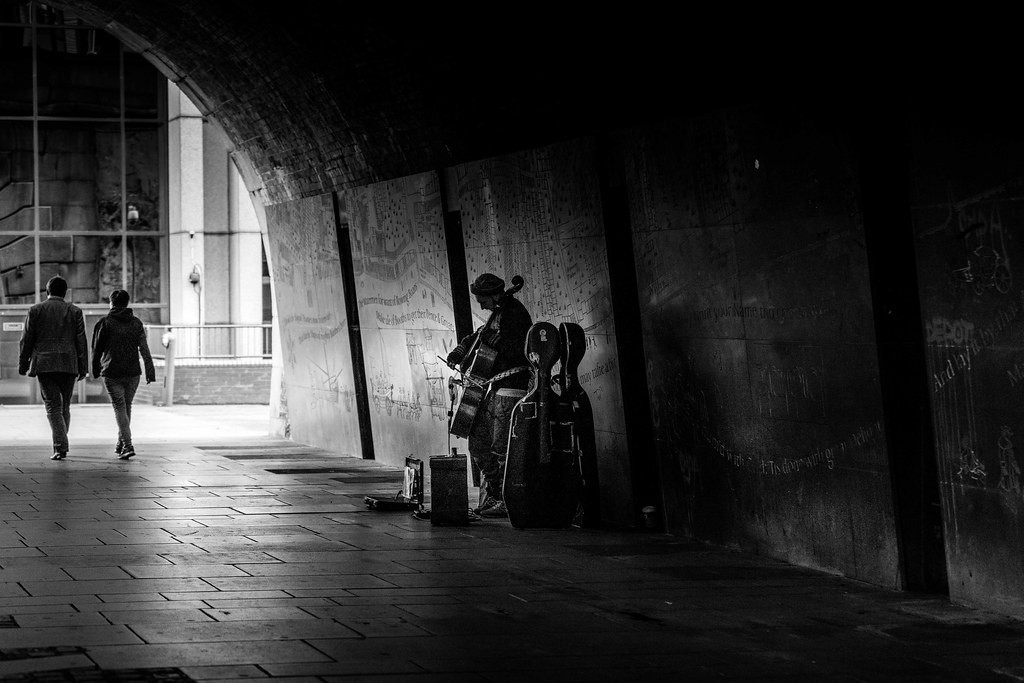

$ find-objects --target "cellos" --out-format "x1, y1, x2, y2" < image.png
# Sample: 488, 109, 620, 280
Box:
447, 274, 525, 439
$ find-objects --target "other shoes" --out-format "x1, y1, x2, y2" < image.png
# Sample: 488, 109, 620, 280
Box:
50, 451, 61, 460
114, 447, 122, 454
117, 445, 135, 459
60, 451, 66, 458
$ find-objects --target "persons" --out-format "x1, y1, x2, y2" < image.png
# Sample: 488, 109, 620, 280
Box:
18, 275, 88, 459
90, 289, 156, 458
446, 273, 533, 518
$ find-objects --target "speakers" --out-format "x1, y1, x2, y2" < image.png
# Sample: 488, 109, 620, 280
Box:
429, 454, 470, 526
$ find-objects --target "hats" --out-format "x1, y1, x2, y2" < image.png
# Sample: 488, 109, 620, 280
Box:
470, 273, 505, 295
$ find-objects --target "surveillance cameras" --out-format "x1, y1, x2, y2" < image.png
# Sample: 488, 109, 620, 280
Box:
128, 210, 139, 225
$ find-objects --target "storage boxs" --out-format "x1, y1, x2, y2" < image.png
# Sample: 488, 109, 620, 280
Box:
429, 454, 468, 525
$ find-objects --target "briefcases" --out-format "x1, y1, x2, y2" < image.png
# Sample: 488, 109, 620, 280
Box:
364, 453, 424, 513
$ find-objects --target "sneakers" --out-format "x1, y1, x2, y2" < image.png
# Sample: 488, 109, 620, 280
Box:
474, 496, 497, 515
479, 500, 509, 518
